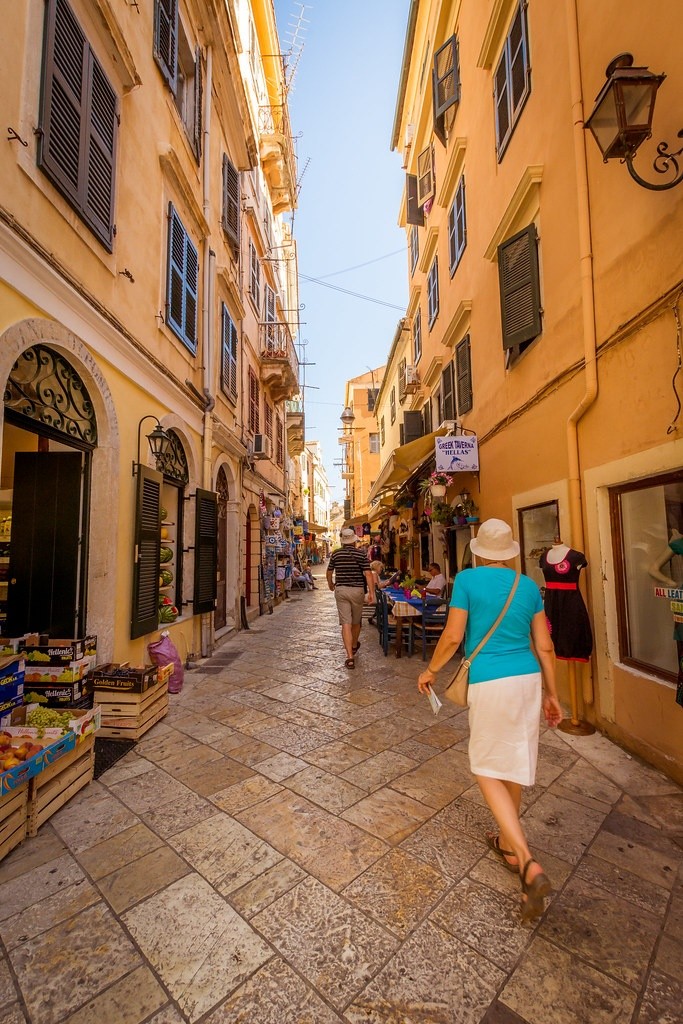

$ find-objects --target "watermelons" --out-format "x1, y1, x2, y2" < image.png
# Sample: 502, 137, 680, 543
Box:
158, 546, 179, 623
161, 506, 168, 520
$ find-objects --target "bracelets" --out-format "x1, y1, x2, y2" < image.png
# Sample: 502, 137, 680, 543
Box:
423, 588, 425, 590
428, 665, 437, 674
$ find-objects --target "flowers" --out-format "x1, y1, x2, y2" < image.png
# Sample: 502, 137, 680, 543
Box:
415, 472, 455, 496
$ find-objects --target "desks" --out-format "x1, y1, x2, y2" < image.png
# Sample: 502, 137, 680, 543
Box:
373, 578, 447, 659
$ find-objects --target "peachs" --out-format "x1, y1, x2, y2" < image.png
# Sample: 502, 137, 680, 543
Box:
0, 730, 55, 776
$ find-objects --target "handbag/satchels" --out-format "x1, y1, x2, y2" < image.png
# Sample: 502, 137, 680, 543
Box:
442, 656, 469, 707
364, 578, 368, 593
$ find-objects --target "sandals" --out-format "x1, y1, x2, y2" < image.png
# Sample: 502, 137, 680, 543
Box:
486, 831, 520, 875
519, 859, 552, 918
353, 641, 361, 655
345, 658, 355, 669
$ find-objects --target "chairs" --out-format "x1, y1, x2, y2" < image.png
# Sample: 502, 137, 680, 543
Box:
291, 573, 304, 592
374, 580, 449, 654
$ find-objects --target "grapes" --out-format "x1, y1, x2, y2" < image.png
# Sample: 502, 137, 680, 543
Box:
108, 667, 139, 677
25, 706, 80, 728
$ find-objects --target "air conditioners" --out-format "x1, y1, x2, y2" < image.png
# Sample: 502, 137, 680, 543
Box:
402, 364, 416, 394
253, 434, 271, 458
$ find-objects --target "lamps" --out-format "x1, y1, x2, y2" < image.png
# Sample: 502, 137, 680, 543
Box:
421, 511, 428, 521
458, 488, 470, 502
411, 517, 418, 526
400, 523, 408, 533
582, 51, 683, 191
392, 527, 398, 535
339, 407, 355, 424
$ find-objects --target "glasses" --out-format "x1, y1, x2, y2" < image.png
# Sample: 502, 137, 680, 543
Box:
429, 568, 434, 572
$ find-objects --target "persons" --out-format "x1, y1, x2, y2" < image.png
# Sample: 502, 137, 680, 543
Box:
327, 529, 374, 669
292, 561, 319, 591
415, 563, 445, 598
648, 538, 683, 659
418, 519, 563, 920
364, 560, 391, 625
538, 542, 593, 663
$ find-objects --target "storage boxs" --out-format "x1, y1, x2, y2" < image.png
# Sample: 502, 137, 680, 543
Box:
0, 635, 158, 797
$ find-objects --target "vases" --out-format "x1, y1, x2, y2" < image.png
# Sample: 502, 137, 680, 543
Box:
430, 485, 445, 497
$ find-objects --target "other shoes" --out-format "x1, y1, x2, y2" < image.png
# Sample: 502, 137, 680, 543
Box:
308, 589, 313, 591
312, 586, 318, 589
368, 617, 374, 625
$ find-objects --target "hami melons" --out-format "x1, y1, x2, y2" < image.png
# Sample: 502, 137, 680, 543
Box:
161, 527, 169, 539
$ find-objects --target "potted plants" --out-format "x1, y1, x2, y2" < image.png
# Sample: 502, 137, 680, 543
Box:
398, 493, 479, 523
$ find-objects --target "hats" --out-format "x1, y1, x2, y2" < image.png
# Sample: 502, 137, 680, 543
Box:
340, 529, 358, 544
468, 519, 521, 561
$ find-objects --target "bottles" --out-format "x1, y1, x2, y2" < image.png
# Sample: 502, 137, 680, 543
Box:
394, 581, 399, 589
422, 588, 426, 599
406, 588, 410, 600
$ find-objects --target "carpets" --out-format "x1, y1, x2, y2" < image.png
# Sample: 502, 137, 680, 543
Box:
92, 739, 136, 780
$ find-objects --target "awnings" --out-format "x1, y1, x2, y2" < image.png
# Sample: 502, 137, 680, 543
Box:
367, 427, 448, 507
